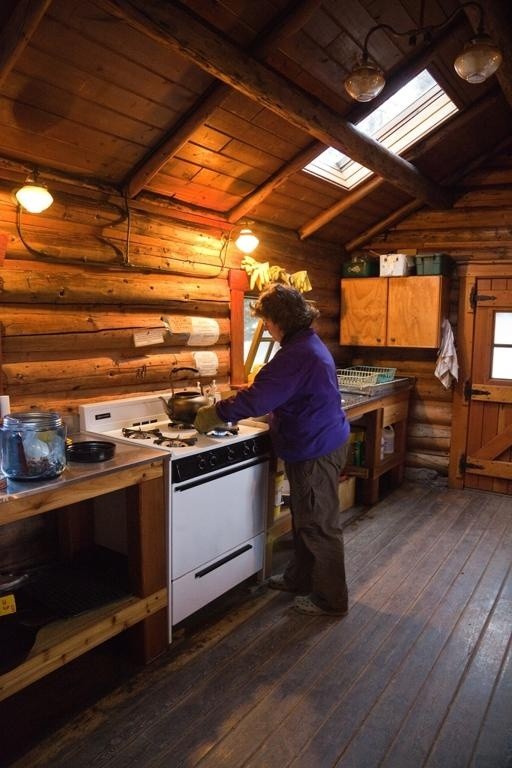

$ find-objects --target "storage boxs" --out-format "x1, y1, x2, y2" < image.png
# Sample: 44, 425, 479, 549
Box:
337, 475, 357, 514
378, 253, 412, 278
414, 253, 455, 275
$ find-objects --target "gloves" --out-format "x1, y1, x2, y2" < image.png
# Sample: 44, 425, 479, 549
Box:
193, 404, 228, 434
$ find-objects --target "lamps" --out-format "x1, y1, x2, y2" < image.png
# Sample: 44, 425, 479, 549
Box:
14, 166, 55, 216
234, 221, 261, 255
341, 0, 506, 105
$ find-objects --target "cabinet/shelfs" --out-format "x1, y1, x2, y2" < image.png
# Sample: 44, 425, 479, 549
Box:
221, 376, 418, 581
1, 429, 170, 704
339, 276, 448, 348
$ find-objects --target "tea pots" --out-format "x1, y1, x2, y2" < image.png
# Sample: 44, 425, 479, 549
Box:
157, 366, 210, 426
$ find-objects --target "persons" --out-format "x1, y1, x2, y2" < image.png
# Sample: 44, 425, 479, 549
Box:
194, 283, 348, 616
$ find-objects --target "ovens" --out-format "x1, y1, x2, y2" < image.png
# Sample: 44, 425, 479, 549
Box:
165, 429, 271, 649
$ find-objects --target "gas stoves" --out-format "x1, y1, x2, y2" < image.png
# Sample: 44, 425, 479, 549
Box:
92, 411, 272, 486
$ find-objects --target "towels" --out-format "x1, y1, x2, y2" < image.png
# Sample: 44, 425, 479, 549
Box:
432, 317, 460, 390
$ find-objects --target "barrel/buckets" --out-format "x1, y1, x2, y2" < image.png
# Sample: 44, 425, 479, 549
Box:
383, 425, 395, 454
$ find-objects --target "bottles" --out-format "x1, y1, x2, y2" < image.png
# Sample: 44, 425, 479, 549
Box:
0, 411, 72, 480
1, 570, 47, 673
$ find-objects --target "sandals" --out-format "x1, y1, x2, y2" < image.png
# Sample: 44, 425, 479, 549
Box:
268, 572, 311, 592
295, 595, 350, 619
